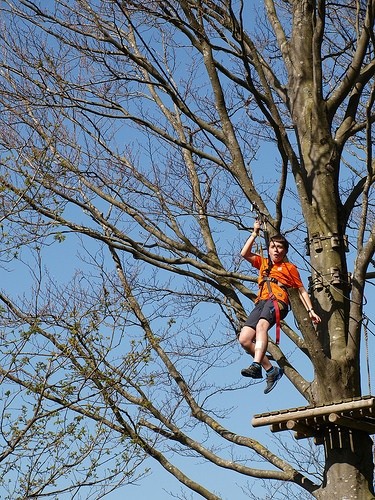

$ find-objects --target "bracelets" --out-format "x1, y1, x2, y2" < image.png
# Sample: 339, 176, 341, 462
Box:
307, 308, 314, 313
252, 232, 258, 238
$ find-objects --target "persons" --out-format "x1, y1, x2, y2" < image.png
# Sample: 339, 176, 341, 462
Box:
238, 219, 322, 394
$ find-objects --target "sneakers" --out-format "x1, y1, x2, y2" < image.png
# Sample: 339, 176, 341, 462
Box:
241, 365, 263, 379
264, 367, 284, 394
